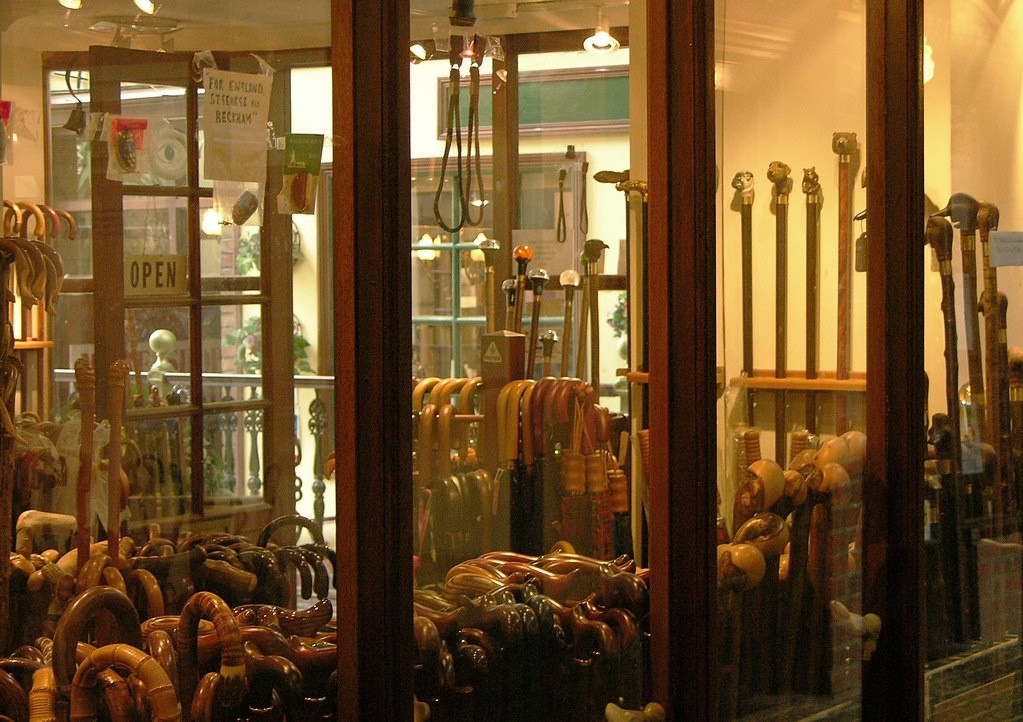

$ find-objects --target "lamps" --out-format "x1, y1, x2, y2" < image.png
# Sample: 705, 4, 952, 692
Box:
57, 0, 83, 10
133, 0, 155, 15
409, 43, 427, 60
583, 6, 621, 58
